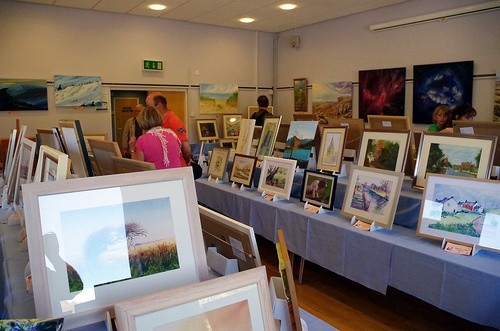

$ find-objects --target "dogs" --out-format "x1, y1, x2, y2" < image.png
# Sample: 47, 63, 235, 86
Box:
306, 179, 328, 198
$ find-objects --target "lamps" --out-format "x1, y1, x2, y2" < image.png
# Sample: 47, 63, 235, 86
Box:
368, 0, 500, 32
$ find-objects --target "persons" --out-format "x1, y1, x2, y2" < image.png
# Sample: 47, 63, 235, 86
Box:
250, 96, 273, 126
146, 92, 192, 166
43, 233, 84, 314
129, 106, 181, 170
428, 105, 476, 133
121, 104, 144, 157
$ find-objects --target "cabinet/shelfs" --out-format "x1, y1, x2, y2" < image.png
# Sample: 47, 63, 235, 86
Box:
194, 151, 500, 331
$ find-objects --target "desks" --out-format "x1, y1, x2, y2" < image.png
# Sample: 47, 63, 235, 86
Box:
0, 189, 118, 331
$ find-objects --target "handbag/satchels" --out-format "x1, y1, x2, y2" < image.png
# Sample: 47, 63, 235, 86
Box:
188, 159, 202, 180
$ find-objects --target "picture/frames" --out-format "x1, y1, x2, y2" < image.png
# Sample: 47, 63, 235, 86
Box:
0, 76, 500, 331
